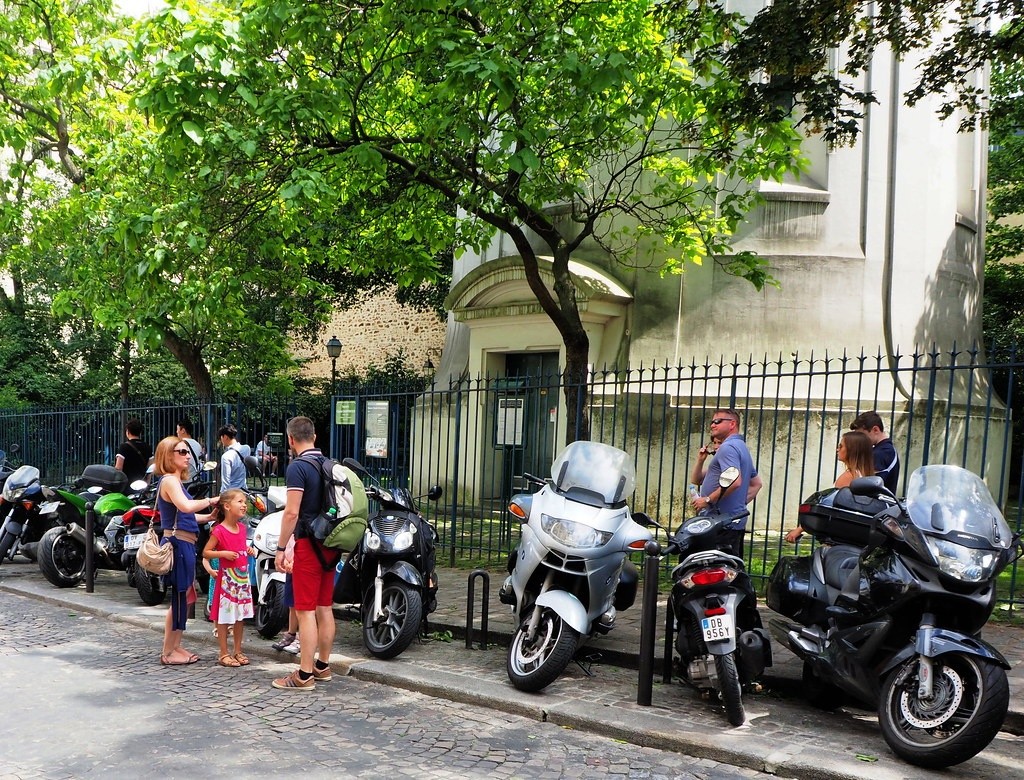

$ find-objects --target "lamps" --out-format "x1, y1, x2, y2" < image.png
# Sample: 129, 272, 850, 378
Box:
423, 347, 442, 377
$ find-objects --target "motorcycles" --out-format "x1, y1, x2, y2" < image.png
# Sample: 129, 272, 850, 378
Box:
498, 440, 655, 694
0, 444, 220, 607
763, 465, 1024, 770
240, 454, 297, 639
629, 466, 775, 725
333, 455, 445, 660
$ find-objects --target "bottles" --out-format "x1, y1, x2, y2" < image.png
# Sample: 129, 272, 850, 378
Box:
689, 483, 709, 516
314, 508, 336, 539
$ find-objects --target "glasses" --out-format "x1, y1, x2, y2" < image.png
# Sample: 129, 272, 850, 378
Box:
173, 449, 190, 457
839, 443, 846, 450
705, 442, 716, 456
710, 418, 733, 425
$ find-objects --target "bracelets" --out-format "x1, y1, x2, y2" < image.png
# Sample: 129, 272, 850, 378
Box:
276, 546, 287, 551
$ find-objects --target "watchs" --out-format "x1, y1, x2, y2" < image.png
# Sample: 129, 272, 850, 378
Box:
705, 496, 711, 504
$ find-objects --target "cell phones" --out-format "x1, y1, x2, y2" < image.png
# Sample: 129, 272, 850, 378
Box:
786, 530, 804, 542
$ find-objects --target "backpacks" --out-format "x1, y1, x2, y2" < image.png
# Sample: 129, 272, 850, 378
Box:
295, 455, 368, 555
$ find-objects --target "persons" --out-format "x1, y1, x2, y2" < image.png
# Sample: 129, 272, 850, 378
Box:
154, 436, 220, 665
691, 408, 761, 558
785, 411, 900, 547
255, 434, 277, 478
201, 489, 257, 666
175, 417, 203, 495
272, 416, 343, 692
218, 424, 252, 522
115, 420, 152, 496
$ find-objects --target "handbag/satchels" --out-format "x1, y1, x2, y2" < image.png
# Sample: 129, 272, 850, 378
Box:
135, 527, 173, 576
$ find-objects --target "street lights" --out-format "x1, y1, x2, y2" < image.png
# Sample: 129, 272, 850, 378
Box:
326, 334, 342, 392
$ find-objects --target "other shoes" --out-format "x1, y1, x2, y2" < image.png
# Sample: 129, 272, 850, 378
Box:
271, 473, 277, 478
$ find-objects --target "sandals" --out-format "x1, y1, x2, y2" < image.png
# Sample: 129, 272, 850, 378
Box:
212, 628, 219, 638
228, 626, 234, 635
160, 653, 198, 666
234, 651, 249, 665
272, 632, 295, 651
175, 646, 200, 660
283, 632, 301, 653
218, 654, 240, 667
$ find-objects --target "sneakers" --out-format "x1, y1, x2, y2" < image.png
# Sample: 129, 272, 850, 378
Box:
313, 661, 332, 681
272, 670, 316, 691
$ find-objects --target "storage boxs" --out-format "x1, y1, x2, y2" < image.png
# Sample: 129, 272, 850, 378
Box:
799, 486, 889, 547
82, 464, 127, 493
